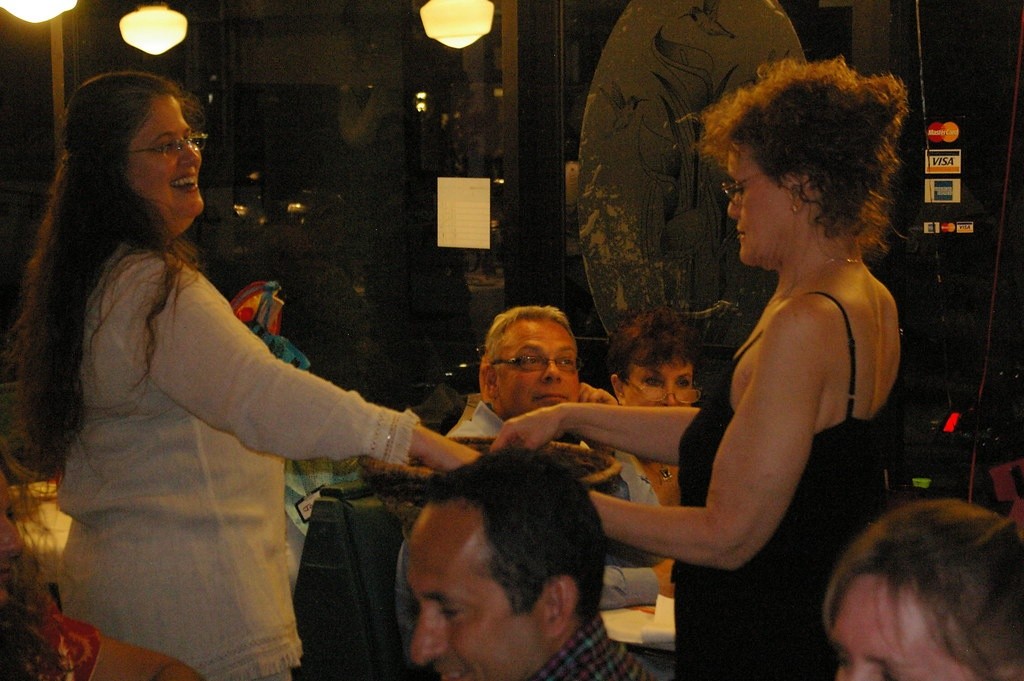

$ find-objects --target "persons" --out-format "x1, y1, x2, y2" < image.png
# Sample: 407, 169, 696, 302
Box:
0, 54, 1024, 680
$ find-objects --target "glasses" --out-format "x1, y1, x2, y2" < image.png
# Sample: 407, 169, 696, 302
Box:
719, 170, 768, 207
625, 378, 703, 404
492, 353, 590, 372
123, 132, 209, 158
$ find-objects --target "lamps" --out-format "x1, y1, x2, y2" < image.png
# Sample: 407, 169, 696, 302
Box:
420, 0, 495, 50
119, 0, 188, 54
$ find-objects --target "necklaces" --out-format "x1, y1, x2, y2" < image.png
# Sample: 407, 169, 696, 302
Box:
658, 461, 672, 480
